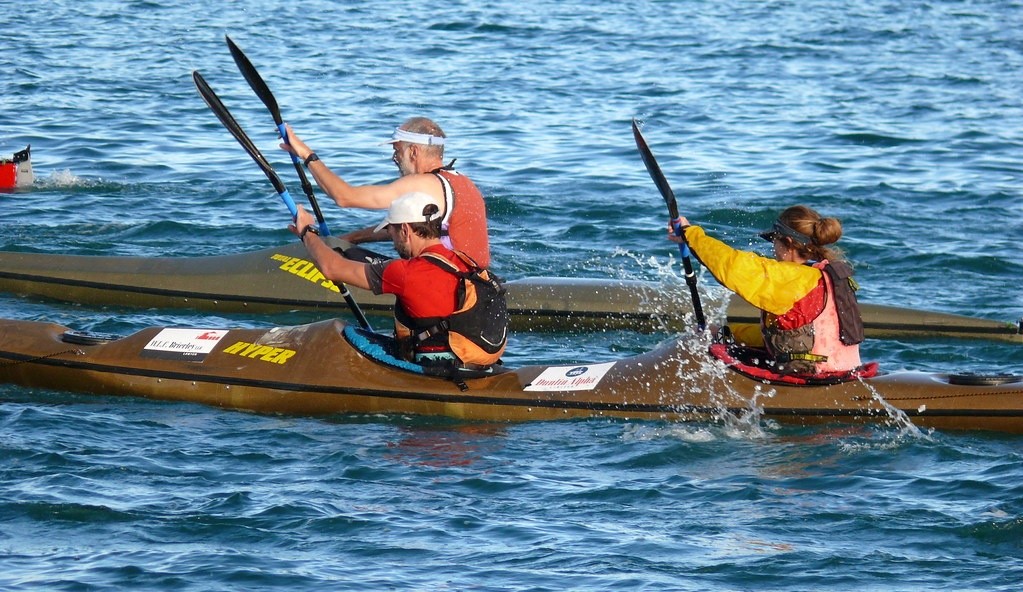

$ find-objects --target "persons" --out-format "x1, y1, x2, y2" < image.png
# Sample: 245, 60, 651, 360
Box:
287, 190, 478, 368
275, 117, 491, 269
666, 205, 861, 382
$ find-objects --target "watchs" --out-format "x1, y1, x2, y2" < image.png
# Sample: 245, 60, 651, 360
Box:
305, 153, 319, 168
300, 225, 320, 242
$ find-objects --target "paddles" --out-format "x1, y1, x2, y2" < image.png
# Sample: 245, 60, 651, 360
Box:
627, 114, 708, 332
192, 67, 377, 336
222, 32, 332, 238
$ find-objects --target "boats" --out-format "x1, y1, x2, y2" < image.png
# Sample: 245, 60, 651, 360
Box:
0, 316, 1023, 435
2, 249, 1023, 339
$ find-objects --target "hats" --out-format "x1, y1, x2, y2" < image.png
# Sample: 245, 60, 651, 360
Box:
371, 192, 443, 233
758, 219, 811, 246
374, 126, 448, 148
